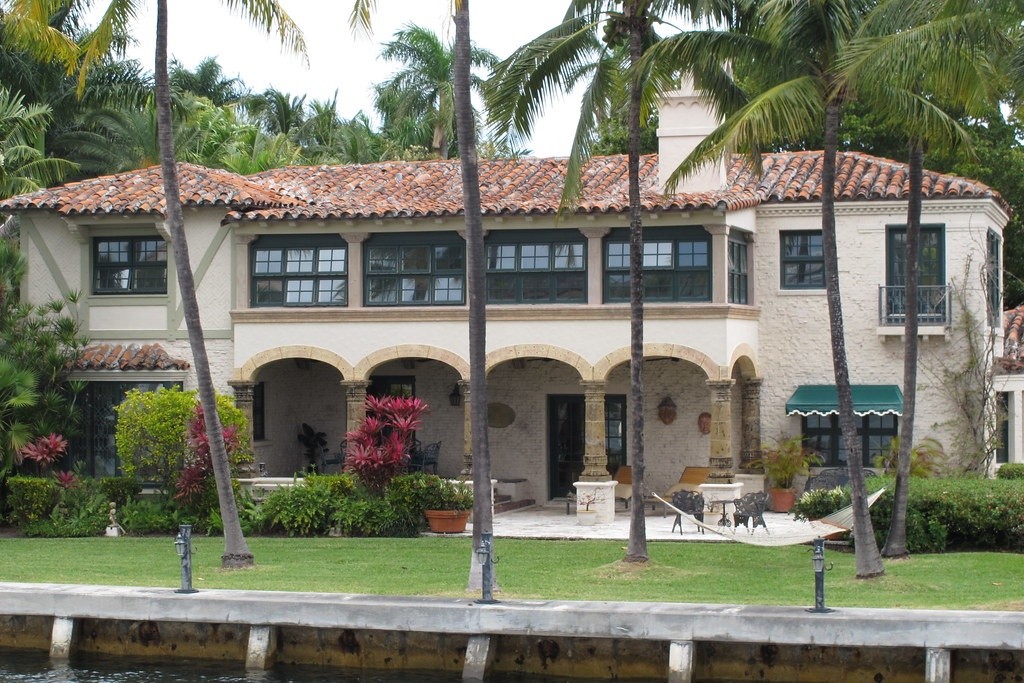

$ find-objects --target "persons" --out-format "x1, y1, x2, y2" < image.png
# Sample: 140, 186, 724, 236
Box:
107, 502, 118, 526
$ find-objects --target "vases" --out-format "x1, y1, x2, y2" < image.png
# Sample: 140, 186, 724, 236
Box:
575, 510, 598, 526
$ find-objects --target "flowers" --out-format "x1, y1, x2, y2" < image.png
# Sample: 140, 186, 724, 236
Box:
567, 486, 608, 511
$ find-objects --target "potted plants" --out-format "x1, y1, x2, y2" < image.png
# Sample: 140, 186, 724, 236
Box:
297, 423, 329, 476
794, 482, 851, 540
742, 428, 826, 512
418, 469, 475, 532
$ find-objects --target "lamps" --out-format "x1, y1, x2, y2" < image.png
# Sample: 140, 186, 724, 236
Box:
449, 383, 461, 406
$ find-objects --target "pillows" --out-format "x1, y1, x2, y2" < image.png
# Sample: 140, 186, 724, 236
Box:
615, 483, 632, 499
664, 483, 699, 497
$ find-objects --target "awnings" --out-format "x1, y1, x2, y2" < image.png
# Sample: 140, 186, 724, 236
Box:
785, 384, 905, 417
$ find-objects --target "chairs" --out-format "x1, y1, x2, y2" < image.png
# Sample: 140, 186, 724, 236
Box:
408, 437, 442, 475
672, 490, 706, 536
567, 465, 646, 514
643, 467, 713, 518
322, 438, 346, 476
734, 490, 771, 537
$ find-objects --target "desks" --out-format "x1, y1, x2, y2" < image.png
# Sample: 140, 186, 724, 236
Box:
710, 500, 734, 536
